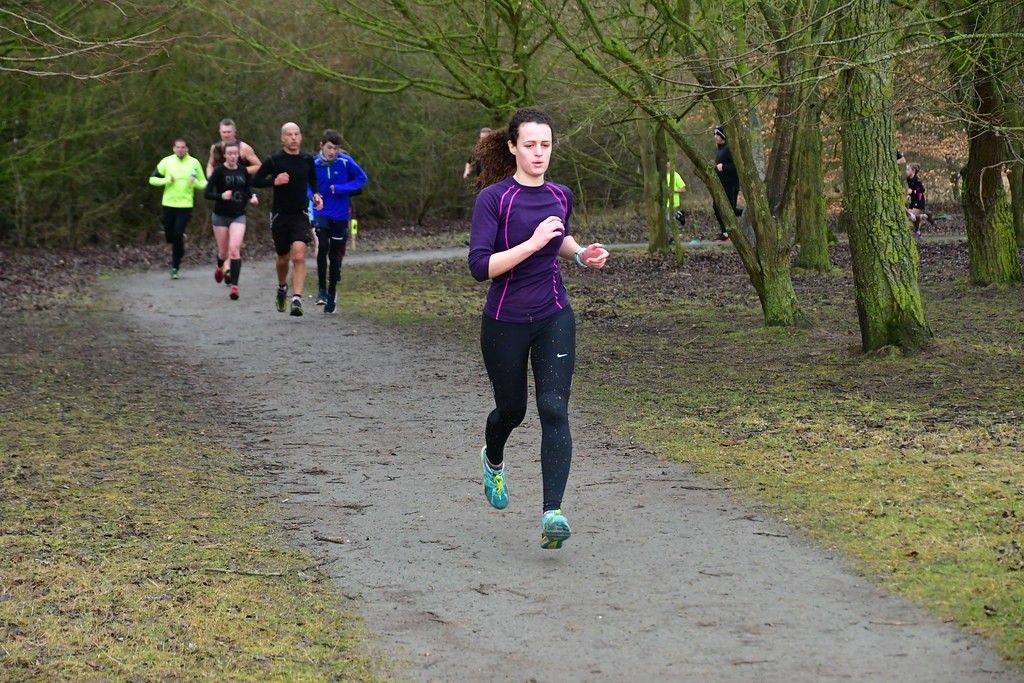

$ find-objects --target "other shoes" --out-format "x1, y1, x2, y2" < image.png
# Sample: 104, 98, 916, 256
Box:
170, 268, 177, 279
926, 211, 933, 225
913, 215, 920, 230
713, 234, 731, 242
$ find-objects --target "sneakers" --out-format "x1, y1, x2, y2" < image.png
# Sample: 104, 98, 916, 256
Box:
316, 289, 327, 305
276, 283, 288, 311
541, 510, 570, 550
290, 299, 304, 316
481, 445, 509, 509
230, 286, 239, 300
677, 209, 685, 226
324, 291, 337, 315
215, 265, 223, 283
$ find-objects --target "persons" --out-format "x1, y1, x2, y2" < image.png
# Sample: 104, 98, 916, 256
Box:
713, 128, 743, 242
666, 160, 686, 244
149, 139, 207, 279
469, 108, 608, 549
252, 122, 323, 315
897, 150, 915, 221
205, 120, 262, 300
464, 128, 493, 179
308, 131, 367, 315
905, 162, 938, 240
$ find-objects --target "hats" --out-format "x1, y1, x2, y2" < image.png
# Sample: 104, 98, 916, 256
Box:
714, 126, 726, 141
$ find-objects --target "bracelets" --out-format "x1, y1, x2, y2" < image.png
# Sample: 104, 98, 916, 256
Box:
574, 249, 589, 270
